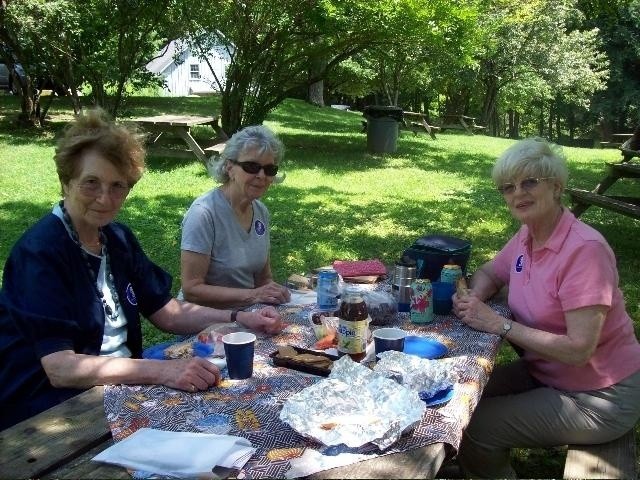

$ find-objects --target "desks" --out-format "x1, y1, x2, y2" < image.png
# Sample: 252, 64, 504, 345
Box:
1, 258, 515, 480
114, 114, 225, 171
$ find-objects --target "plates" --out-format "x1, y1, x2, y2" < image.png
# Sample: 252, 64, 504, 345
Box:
416, 385, 455, 408
269, 345, 340, 376
400, 335, 448, 362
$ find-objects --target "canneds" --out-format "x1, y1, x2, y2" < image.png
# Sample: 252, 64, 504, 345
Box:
410, 278, 434, 325
316, 269, 338, 309
440, 264, 462, 284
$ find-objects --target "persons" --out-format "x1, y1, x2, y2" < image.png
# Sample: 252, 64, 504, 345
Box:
1, 111, 283, 431
176, 125, 291, 307
451, 136, 640, 479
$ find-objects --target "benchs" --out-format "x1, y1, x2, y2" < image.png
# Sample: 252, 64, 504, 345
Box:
358, 105, 488, 140
565, 121, 640, 224
204, 139, 229, 161
559, 425, 639, 480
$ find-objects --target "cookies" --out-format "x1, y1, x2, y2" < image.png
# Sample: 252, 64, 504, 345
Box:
274, 345, 332, 372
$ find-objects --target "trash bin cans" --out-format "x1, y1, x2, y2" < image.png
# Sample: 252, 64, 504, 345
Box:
365, 107, 402, 154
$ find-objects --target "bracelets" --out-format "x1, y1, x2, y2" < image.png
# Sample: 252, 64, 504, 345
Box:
231, 308, 245, 323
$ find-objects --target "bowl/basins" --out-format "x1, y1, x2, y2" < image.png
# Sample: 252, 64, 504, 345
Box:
430, 281, 456, 302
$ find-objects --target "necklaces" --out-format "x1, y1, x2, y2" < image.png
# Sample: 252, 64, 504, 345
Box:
86, 241, 101, 248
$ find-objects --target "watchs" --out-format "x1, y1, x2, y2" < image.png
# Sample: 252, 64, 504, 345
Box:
500, 321, 510, 337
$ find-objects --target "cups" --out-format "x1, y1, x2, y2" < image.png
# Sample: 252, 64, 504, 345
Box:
220, 332, 257, 379
372, 327, 406, 361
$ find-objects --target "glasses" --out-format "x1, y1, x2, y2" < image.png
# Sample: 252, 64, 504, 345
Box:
229, 158, 278, 176
498, 176, 556, 194
71, 179, 131, 198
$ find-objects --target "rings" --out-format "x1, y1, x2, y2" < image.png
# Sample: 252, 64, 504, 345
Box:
189, 384, 194, 393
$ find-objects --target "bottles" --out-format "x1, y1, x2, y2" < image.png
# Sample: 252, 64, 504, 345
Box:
392, 254, 418, 312
337, 288, 368, 361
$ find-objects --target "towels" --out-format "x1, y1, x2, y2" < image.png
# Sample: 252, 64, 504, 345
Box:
330, 255, 388, 278
